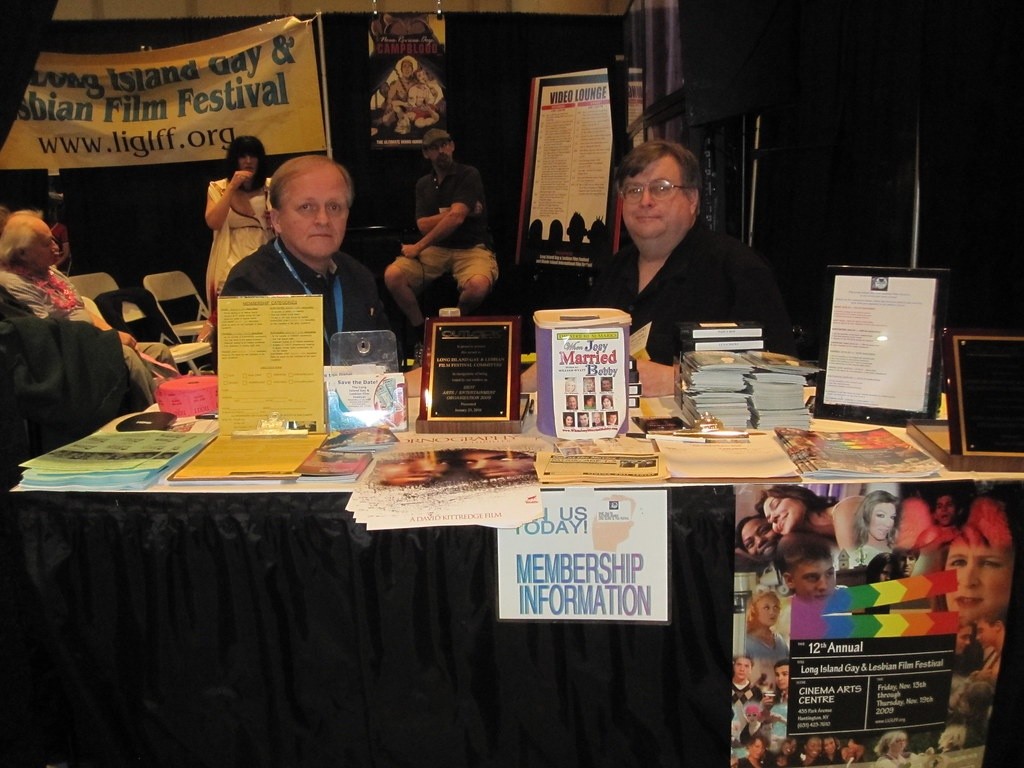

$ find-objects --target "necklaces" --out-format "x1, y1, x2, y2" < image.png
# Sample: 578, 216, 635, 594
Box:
6, 267, 76, 309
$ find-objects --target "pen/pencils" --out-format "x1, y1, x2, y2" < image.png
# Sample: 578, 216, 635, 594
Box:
625, 431, 708, 445
370, 306, 375, 316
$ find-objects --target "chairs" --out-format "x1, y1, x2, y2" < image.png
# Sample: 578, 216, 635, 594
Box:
69, 272, 214, 378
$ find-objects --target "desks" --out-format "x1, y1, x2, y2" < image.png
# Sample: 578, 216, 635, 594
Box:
346, 226, 414, 374
0, 405, 1024, 768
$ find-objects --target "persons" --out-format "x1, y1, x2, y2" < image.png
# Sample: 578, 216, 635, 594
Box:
209, 155, 424, 397
564, 378, 618, 427
520, 142, 747, 397
732, 487, 1015, 768
372, 448, 538, 488
205, 136, 277, 312
0, 198, 180, 407
384, 130, 499, 370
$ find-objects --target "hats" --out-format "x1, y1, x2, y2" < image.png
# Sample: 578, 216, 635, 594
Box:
421, 129, 450, 149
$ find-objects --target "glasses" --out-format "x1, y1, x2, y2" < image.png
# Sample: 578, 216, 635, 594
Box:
617, 179, 690, 204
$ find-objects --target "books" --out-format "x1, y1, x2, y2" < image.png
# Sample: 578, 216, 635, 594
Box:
679, 353, 826, 431
294, 425, 400, 482
774, 427, 944, 478
